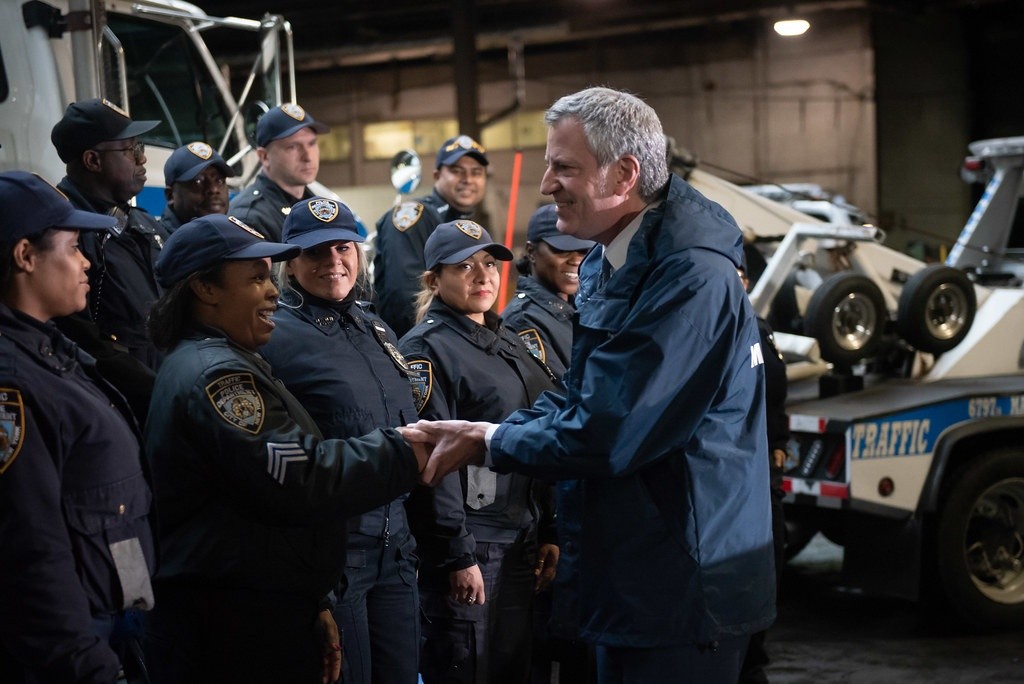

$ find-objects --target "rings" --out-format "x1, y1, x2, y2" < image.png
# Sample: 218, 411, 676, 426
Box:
539, 560, 544, 563
468, 594, 477, 602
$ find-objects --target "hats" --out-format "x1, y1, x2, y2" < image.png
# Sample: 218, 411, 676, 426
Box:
424, 220, 514, 270
255, 103, 322, 146
164, 142, 235, 186
282, 197, 365, 250
51, 99, 162, 163
527, 204, 597, 250
155, 213, 303, 289
0, 171, 119, 242
437, 135, 488, 167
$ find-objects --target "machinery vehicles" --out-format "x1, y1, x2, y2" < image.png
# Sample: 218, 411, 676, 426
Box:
664, 132, 1024, 628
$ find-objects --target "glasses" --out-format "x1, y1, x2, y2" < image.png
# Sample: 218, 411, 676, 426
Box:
80, 141, 144, 159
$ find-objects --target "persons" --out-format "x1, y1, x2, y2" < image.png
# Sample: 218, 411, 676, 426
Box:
1, 171, 154, 684
397, 219, 565, 684
499, 203, 598, 395
374, 135, 494, 343
737, 236, 790, 684
257, 195, 421, 683
226, 102, 331, 245
51, 98, 177, 375
140, 212, 437, 684
418, 84, 779, 684
155, 141, 229, 242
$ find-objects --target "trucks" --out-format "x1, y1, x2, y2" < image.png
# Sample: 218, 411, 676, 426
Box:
0, 1, 421, 227
